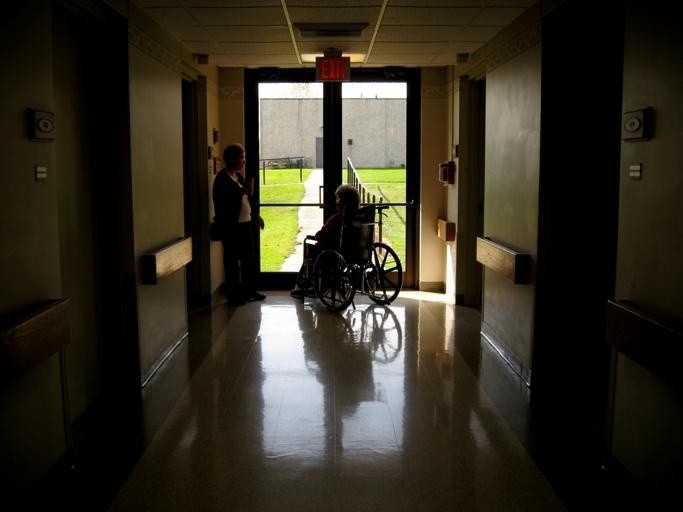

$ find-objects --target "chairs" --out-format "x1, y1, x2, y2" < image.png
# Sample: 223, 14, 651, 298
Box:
244, 293, 265, 300
303, 288, 317, 297
290, 288, 302, 299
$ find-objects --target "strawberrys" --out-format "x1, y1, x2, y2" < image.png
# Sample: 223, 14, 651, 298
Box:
298, 205, 404, 311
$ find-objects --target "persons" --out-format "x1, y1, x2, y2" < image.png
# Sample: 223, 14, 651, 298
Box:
213, 144, 266, 305
291, 185, 361, 299
